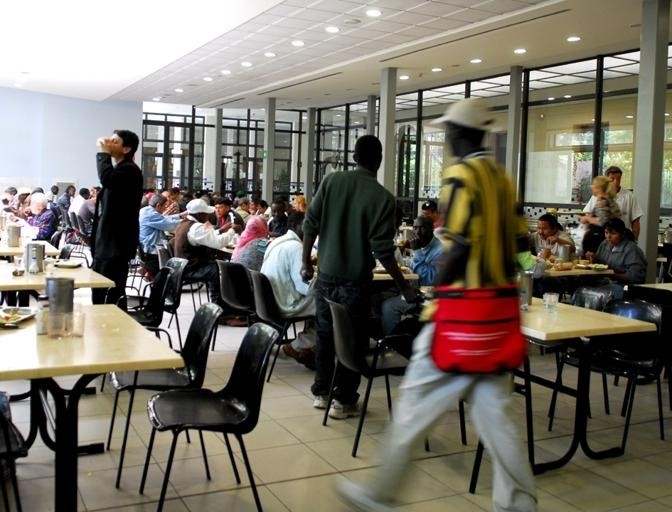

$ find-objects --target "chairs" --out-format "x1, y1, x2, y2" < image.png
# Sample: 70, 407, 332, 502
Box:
139, 322, 279, 512
156, 241, 209, 329
211, 258, 316, 382
105, 302, 222, 489
0, 199, 189, 392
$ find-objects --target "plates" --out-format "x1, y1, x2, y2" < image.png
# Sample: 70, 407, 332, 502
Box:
53, 258, 85, 269
0, 305, 38, 328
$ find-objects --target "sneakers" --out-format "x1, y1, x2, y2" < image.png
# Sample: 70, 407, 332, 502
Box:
314, 395, 363, 419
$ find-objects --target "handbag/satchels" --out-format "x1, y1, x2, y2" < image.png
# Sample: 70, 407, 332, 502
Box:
431, 285, 527, 374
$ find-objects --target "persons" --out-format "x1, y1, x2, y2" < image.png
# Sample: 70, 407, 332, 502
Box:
4, 185, 102, 248
516, 167, 648, 319
90, 128, 144, 315
374, 199, 442, 344
332, 97, 540, 512
137, 188, 317, 373
299, 133, 414, 419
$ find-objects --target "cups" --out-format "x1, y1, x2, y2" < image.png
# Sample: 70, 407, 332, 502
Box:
517, 269, 560, 316
45, 275, 86, 339
536, 242, 609, 271
6, 224, 24, 249
96, 137, 107, 146
24, 243, 45, 276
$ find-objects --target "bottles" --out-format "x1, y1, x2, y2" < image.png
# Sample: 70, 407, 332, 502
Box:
37, 295, 51, 335
12, 256, 56, 276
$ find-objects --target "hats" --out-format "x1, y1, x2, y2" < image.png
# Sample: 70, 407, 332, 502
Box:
429, 98, 496, 131
186, 199, 215, 214
